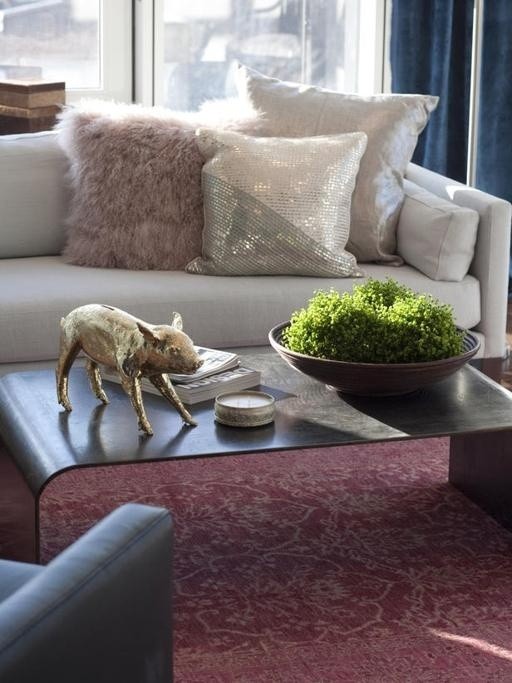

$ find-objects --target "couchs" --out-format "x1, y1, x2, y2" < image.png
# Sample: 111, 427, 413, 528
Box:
1, 129, 511, 383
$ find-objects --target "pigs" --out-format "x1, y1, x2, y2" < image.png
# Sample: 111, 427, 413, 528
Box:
55, 303, 204, 436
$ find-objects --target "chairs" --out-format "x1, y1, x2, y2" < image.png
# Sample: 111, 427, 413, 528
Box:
0, 501, 174, 683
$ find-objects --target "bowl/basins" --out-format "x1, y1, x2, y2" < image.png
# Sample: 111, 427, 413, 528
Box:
267, 322, 481, 395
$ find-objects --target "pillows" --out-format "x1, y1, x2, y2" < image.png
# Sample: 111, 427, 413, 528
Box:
183, 125, 368, 277
233, 56, 442, 268
53, 99, 270, 271
395, 177, 479, 282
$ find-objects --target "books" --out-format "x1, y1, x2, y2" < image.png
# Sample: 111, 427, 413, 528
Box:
101, 345, 262, 405
